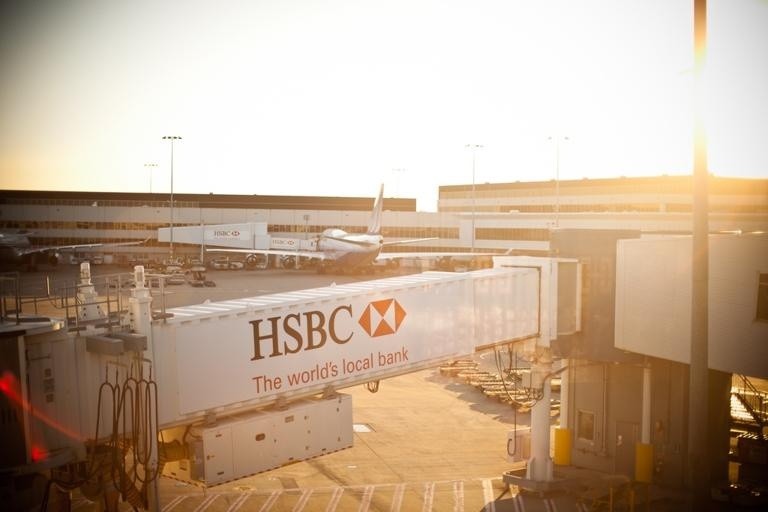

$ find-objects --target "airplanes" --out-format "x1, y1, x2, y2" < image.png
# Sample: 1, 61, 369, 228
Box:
204, 182, 513, 276
0, 229, 150, 274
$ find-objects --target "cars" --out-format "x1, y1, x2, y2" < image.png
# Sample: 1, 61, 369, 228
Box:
210, 255, 266, 269
70, 255, 103, 265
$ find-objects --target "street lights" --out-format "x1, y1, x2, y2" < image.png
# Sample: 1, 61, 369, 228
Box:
544, 136, 571, 243
464, 140, 486, 257
162, 134, 183, 263
141, 161, 160, 194
